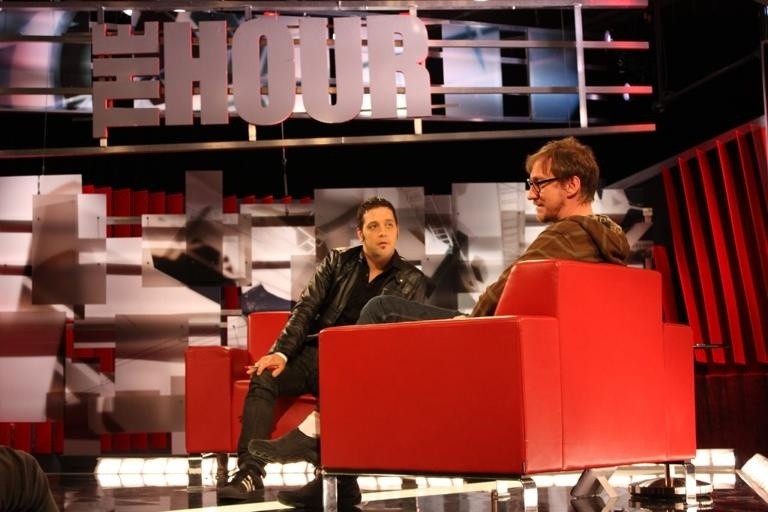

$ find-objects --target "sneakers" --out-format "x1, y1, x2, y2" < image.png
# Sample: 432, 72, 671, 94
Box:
247, 426, 321, 466
276, 473, 365, 510
215, 469, 266, 501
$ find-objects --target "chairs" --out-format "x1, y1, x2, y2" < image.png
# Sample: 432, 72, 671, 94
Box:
184, 310, 316, 481
319, 256, 697, 511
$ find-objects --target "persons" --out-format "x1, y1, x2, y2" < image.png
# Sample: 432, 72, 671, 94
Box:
0, 443, 61, 511
217, 197, 430, 501
249, 135, 632, 509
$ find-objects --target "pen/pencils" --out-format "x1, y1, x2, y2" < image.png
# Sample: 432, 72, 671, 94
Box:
243, 366, 278, 370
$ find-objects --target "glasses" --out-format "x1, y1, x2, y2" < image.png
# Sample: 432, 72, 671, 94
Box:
526, 177, 560, 193
